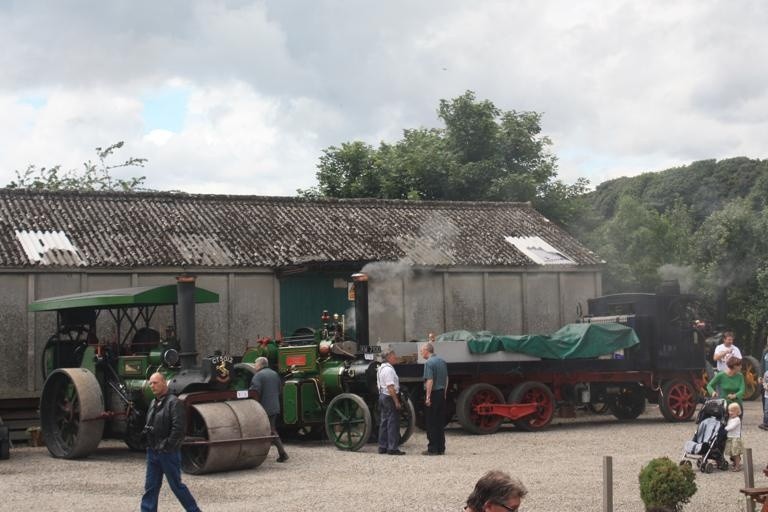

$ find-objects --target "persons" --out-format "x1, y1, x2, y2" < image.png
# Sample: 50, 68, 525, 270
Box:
463, 468, 528, 512
712, 331, 742, 397
375, 347, 406, 455
705, 356, 745, 419
248, 356, 289, 462
757, 370, 768, 431
419, 342, 449, 455
720, 401, 742, 472
138, 373, 202, 511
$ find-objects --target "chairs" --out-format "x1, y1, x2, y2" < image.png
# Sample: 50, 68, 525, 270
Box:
130, 328, 160, 358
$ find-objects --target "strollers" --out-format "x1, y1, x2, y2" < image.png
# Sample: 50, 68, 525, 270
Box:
679, 392, 734, 474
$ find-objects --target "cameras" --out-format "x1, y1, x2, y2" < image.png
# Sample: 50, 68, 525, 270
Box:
143, 425, 154, 434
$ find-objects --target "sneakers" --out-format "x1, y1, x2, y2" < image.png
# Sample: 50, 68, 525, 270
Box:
420, 448, 445, 456
277, 451, 290, 464
377, 446, 407, 456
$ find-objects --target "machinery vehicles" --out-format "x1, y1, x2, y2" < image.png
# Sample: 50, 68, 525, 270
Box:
230, 271, 417, 452
28, 277, 279, 476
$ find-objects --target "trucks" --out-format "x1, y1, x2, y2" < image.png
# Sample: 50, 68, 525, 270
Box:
365, 293, 706, 436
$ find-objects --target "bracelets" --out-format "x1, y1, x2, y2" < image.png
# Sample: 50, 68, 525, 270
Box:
427, 396, 430, 400
